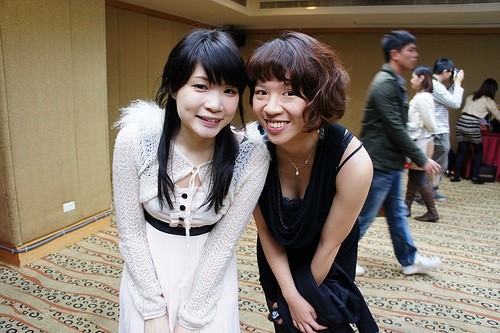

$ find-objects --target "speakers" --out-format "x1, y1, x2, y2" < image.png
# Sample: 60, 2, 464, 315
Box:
231, 32, 247, 47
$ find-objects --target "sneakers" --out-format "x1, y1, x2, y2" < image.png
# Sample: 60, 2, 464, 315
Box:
402, 256, 440, 275
355, 265, 364, 275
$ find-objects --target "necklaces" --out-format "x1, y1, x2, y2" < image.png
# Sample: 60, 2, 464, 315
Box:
283, 148, 314, 175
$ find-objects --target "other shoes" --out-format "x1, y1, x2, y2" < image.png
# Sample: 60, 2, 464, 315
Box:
472, 177, 484, 183
415, 192, 446, 207
450, 176, 460, 181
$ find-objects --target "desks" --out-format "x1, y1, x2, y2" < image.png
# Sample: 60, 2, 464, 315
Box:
462, 129, 500, 182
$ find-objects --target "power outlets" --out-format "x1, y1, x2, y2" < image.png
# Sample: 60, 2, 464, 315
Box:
62, 200, 76, 213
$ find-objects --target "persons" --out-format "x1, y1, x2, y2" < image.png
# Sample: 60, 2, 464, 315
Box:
404, 64, 441, 222
413, 58, 465, 207
252, 29, 382, 333
355, 30, 442, 274
111, 29, 269, 333
450, 78, 500, 184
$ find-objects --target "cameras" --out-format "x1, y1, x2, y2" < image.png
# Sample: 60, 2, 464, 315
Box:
271, 308, 280, 320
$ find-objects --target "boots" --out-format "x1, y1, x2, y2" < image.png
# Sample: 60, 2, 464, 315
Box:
406, 182, 418, 216
415, 186, 439, 221
